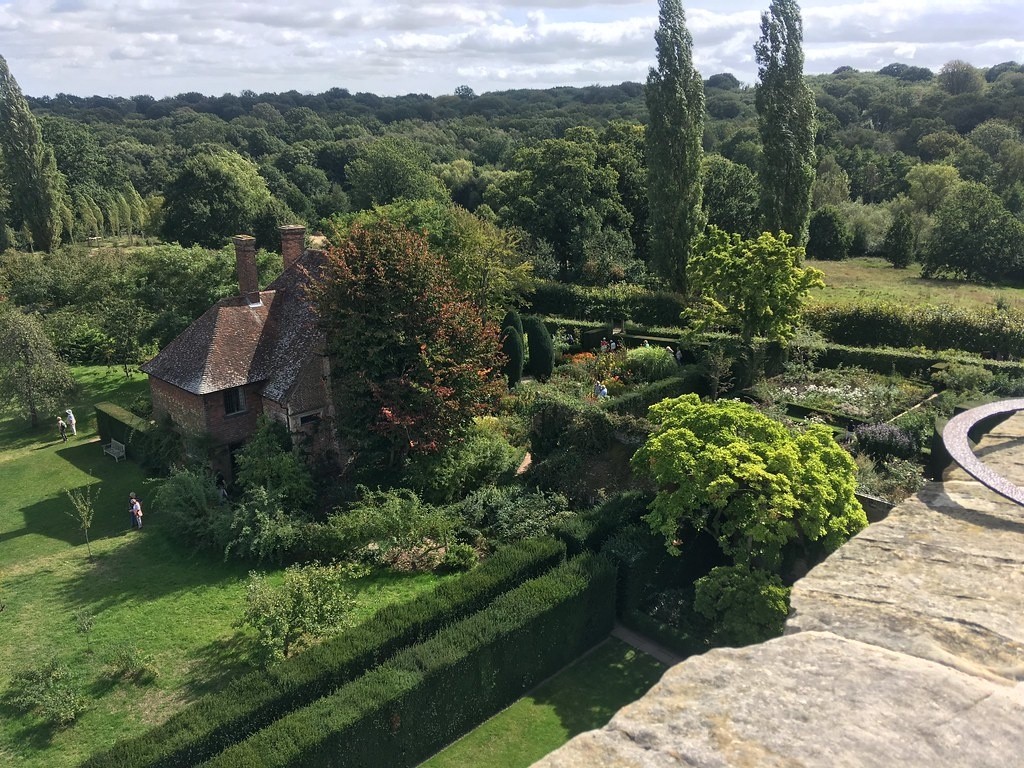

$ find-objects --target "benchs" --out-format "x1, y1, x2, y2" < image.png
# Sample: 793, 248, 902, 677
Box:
102, 438, 127, 463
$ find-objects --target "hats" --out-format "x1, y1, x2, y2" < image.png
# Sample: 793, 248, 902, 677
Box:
56, 417, 62, 420
66, 410, 72, 414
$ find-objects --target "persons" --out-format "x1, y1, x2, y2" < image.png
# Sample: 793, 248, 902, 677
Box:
129, 492, 143, 529
564, 335, 684, 397
216, 469, 227, 498
65, 410, 77, 435
57, 417, 68, 442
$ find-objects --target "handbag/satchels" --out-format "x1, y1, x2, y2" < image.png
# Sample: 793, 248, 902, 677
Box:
61, 422, 65, 431
137, 509, 143, 516
